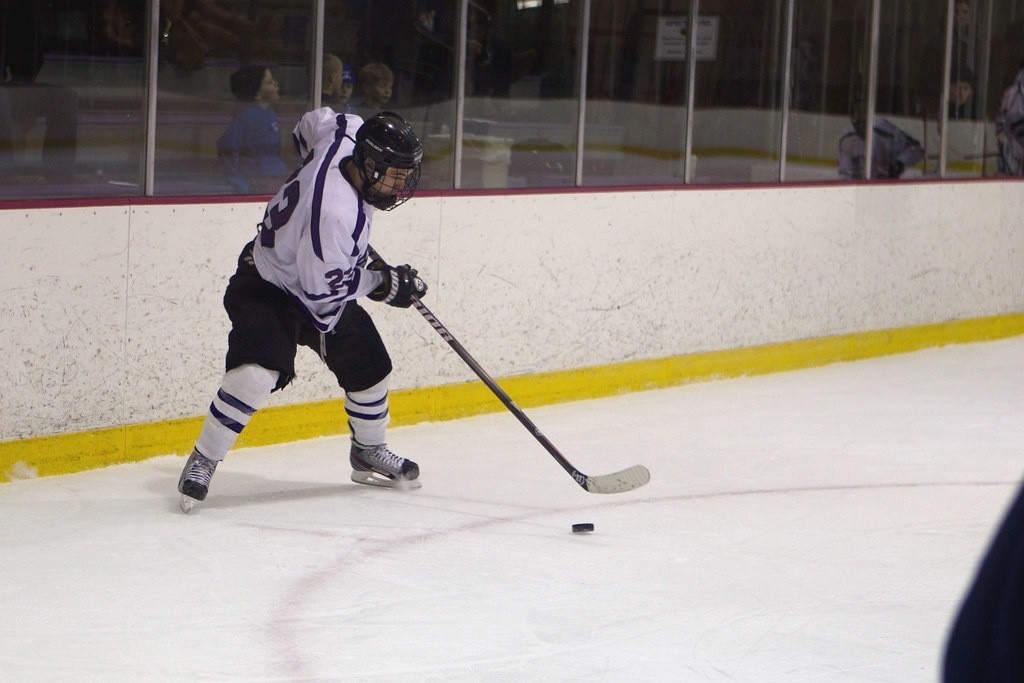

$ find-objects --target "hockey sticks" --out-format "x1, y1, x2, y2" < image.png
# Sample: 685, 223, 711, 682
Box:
363, 241, 651, 496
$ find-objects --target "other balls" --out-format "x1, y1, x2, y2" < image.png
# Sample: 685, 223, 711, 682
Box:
571, 523, 595, 533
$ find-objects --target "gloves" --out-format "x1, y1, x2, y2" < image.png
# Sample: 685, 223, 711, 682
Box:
367, 259, 428, 308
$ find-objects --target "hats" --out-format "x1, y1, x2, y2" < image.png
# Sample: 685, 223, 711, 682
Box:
342, 70, 352, 83
230, 65, 265, 103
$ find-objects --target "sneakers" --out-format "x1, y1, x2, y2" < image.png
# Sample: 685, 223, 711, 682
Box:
347, 419, 423, 488
177, 446, 219, 513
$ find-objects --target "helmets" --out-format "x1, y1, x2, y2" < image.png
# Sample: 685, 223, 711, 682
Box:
352, 110, 424, 212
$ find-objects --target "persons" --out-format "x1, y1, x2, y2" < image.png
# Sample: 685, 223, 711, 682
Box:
938, 63, 977, 120
354, 62, 394, 123
178, 105, 429, 515
214, 63, 298, 196
321, 52, 353, 114
